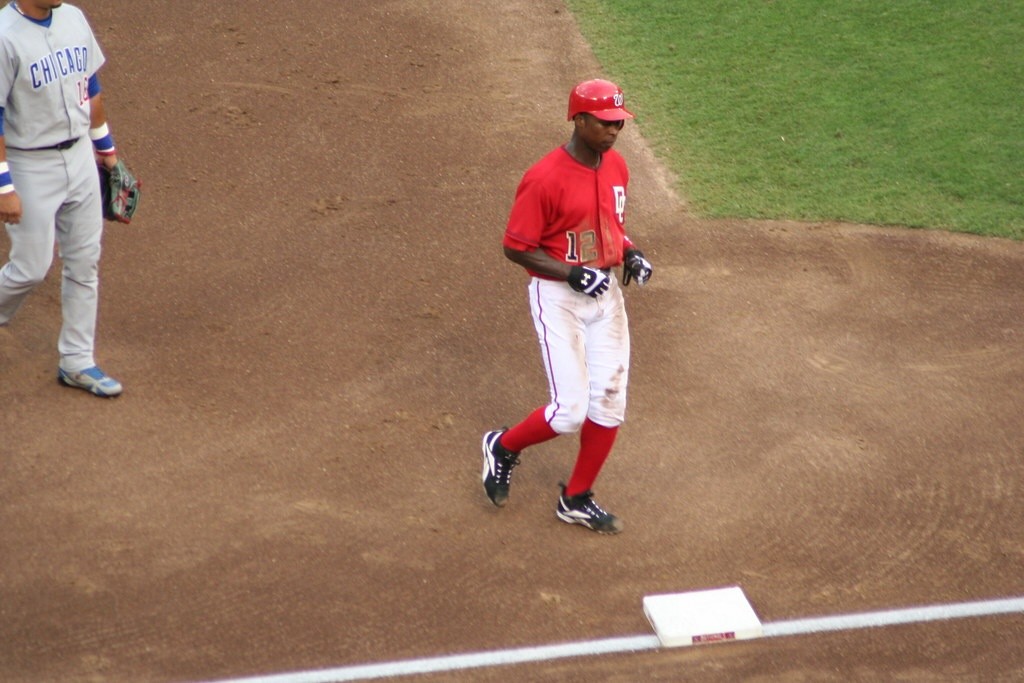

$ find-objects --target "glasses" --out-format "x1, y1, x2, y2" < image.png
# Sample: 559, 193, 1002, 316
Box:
597, 119, 624, 130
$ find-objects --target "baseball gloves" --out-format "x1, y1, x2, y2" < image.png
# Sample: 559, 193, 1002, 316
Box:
97, 157, 144, 227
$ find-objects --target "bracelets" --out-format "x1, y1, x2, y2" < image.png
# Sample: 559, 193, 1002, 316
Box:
94, 150, 115, 156
0, 162, 15, 194
90, 123, 115, 151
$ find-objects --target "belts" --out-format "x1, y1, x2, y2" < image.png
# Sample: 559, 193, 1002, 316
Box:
5, 137, 80, 152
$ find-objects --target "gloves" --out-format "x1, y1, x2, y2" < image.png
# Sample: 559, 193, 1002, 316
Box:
622, 249, 653, 288
565, 266, 610, 298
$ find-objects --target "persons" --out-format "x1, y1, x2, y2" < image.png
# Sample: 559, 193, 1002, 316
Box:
0, 0, 123, 397
481, 79, 652, 535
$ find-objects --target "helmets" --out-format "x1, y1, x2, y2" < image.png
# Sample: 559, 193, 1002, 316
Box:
567, 78, 636, 121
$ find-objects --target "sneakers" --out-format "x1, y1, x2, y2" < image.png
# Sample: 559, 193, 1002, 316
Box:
57, 363, 123, 397
479, 425, 520, 510
554, 482, 625, 535
